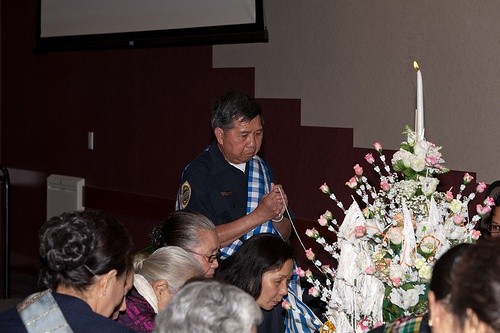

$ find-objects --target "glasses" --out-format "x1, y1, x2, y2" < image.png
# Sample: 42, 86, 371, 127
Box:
184, 248, 221, 263
487, 224, 500, 233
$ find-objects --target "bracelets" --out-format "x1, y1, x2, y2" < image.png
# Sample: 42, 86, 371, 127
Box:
272, 215, 283, 222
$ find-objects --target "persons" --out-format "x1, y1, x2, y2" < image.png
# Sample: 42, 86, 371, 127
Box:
175, 94, 323, 333
115, 246, 203, 333
215, 233, 296, 333
366, 180, 500, 333
153, 282, 263, 333
139, 210, 220, 280
0, 211, 134, 333
